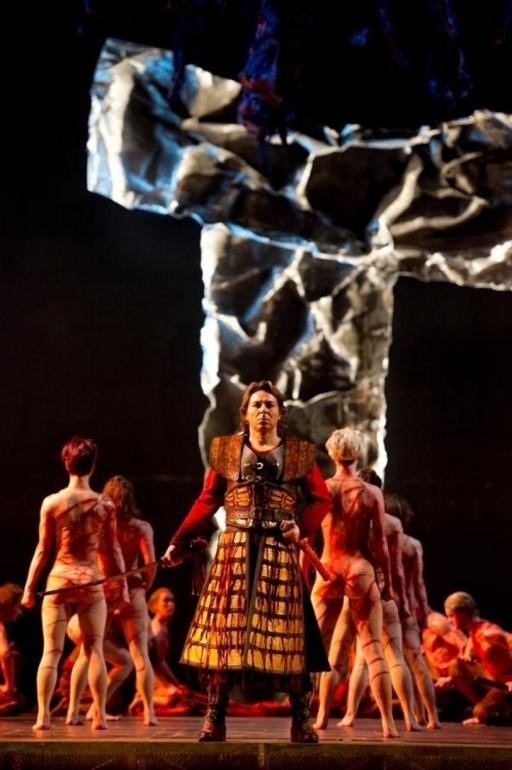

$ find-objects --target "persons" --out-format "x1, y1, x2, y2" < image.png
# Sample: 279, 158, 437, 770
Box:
335, 495, 447, 734
19, 435, 131, 728
0, 583, 512, 729
65, 473, 162, 728
160, 380, 334, 745
300, 422, 404, 740
308, 468, 427, 733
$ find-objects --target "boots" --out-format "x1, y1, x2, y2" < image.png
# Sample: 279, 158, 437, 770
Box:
285, 671, 318, 743
198, 670, 235, 741
